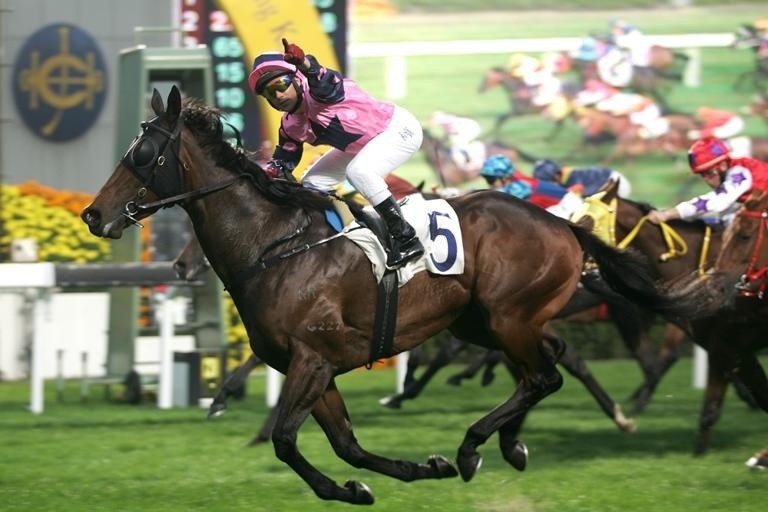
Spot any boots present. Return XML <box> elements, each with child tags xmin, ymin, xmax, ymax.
<box><xmin>373</xmin><ymin>195</ymin><xmax>424</xmax><ymax>267</ymax></box>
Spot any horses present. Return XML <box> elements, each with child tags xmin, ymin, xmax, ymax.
<box><xmin>477</xmin><ymin>32</ymin><xmax>701</xmax><ymax>166</ymax></box>
<box><xmin>731</xmin><ymin>23</ymin><xmax>768</xmax><ymax>90</ymax></box>
<box><xmin>169</xmin><ymin>230</ymin><xmax>638</xmax><ymax>452</ymax></box>
<box><xmin>739</xmin><ymin>92</ymin><xmax>768</xmax><ymax>128</ymax></box>
<box><xmin>666</xmin><ymin>122</ymin><xmax>767</xmax><ymax>166</ymax></box>
<box><xmin>380</xmin><ymin>178</ymin><xmax>768</xmax><ymax>475</ymax></box>
<box><xmin>79</xmin><ymin>83</ymin><xmax>698</xmax><ymax>506</ymax></box>
<box><xmin>418</xmin><ymin>129</ymin><xmax>531</xmax><ymax>193</ymax></box>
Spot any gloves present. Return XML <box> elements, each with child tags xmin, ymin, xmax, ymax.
<box><xmin>281</xmin><ymin>39</ymin><xmax>307</xmax><ymax>65</ymax></box>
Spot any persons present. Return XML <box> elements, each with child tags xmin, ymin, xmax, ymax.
<box><xmin>647</xmin><ymin>135</ymin><xmax>768</xmax><ymax>225</ymax></box>
<box><xmin>532</xmin><ymin>158</ymin><xmax>632</xmax><ymax>199</ymax></box>
<box><xmin>477</xmin><ymin>154</ymin><xmax>588</xmax><ymax>226</ymax></box>
<box><xmin>247</xmin><ymin>38</ymin><xmax>425</xmax><ymax>266</ymax></box>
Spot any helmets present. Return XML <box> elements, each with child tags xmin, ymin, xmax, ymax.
<box><xmin>688</xmin><ymin>135</ymin><xmax>730</xmax><ymax>176</ymax></box>
<box><xmin>247</xmin><ymin>51</ymin><xmax>304</xmax><ymax>90</ymax></box>
<box><xmin>479</xmin><ymin>153</ymin><xmax>515</xmax><ymax>178</ymax></box>
<box><xmin>532</xmin><ymin>158</ymin><xmax>561</xmax><ymax>182</ymax></box>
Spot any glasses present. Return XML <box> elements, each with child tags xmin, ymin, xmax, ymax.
<box><xmin>260</xmin><ymin>74</ymin><xmax>294</xmax><ymax>101</ymax></box>
